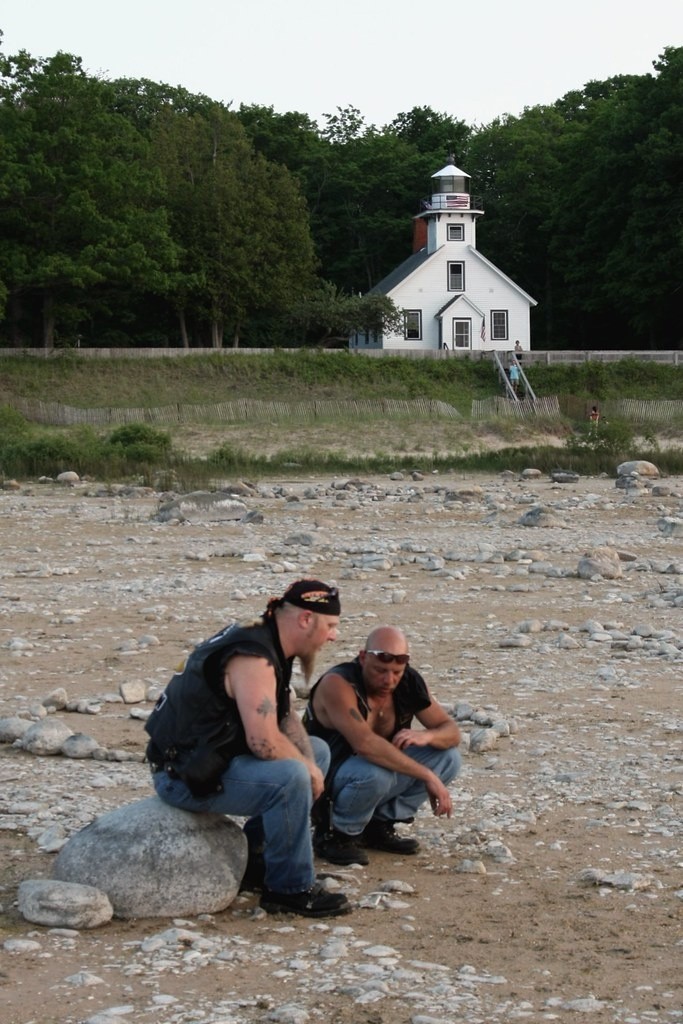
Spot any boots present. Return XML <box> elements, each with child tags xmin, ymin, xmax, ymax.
<box><xmin>351</xmin><ymin>815</ymin><xmax>421</xmax><ymax>852</ymax></box>
<box><xmin>313</xmin><ymin>827</ymin><xmax>371</xmax><ymax>865</ymax></box>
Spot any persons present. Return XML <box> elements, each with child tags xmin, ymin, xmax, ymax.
<box><xmin>141</xmin><ymin>578</ymin><xmax>352</xmax><ymax>919</ymax></box>
<box><xmin>301</xmin><ymin>625</ymin><xmax>463</xmax><ymax>867</ymax></box>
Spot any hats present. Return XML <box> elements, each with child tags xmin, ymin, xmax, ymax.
<box><xmin>285</xmin><ymin>580</ymin><xmax>341</xmax><ymax>617</ymax></box>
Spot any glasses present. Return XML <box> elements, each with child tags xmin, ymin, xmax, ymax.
<box><xmin>364</xmin><ymin>649</ymin><xmax>410</xmax><ymax>665</ymax></box>
<box><xmin>315</xmin><ymin>586</ymin><xmax>340</xmax><ymax>605</ymax></box>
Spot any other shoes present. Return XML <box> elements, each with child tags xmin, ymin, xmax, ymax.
<box><xmin>239</xmin><ymin>870</ymin><xmax>267</xmax><ymax>894</ymax></box>
<box><xmin>259</xmin><ymin>883</ymin><xmax>350</xmax><ymax>916</ymax></box>
